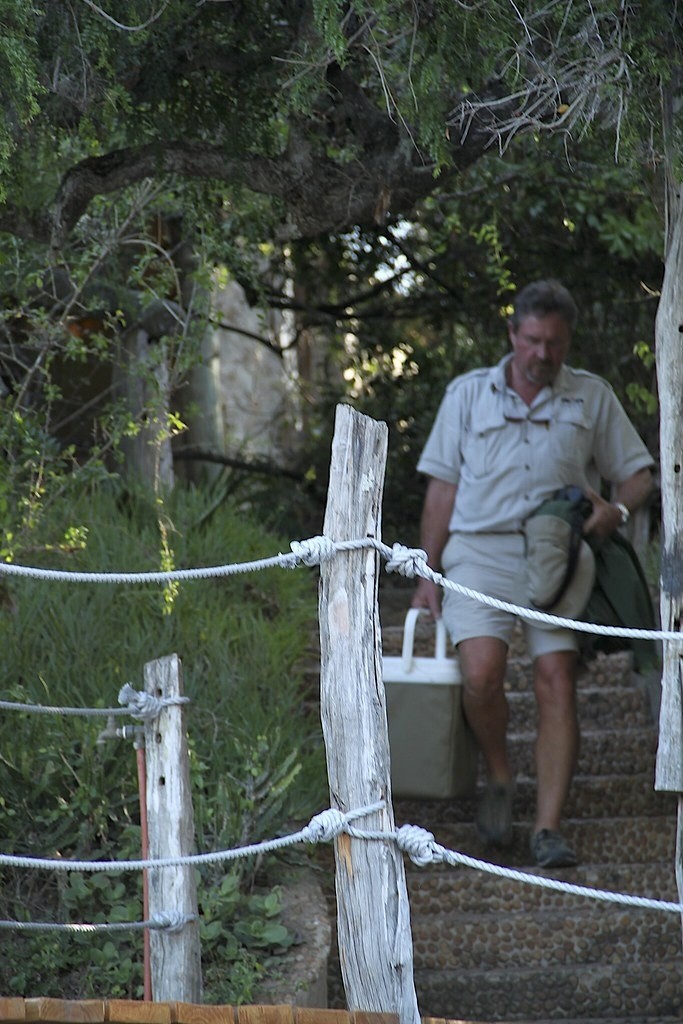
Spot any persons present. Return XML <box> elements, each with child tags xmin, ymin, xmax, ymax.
<box><xmin>410</xmin><ymin>274</ymin><xmax>655</xmax><ymax>872</ymax></box>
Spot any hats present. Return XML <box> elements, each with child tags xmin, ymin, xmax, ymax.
<box><xmin>520</xmin><ymin>515</ymin><xmax>595</xmax><ymax>630</ymax></box>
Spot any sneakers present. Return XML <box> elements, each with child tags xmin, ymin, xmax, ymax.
<box><xmin>476</xmin><ymin>774</ymin><xmax>516</xmax><ymax>851</ymax></box>
<box><xmin>529</xmin><ymin>829</ymin><xmax>576</xmax><ymax>867</ymax></box>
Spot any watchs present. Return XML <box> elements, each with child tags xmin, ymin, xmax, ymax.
<box><xmin>616</xmin><ymin>501</ymin><xmax>633</xmax><ymax>525</ymax></box>
